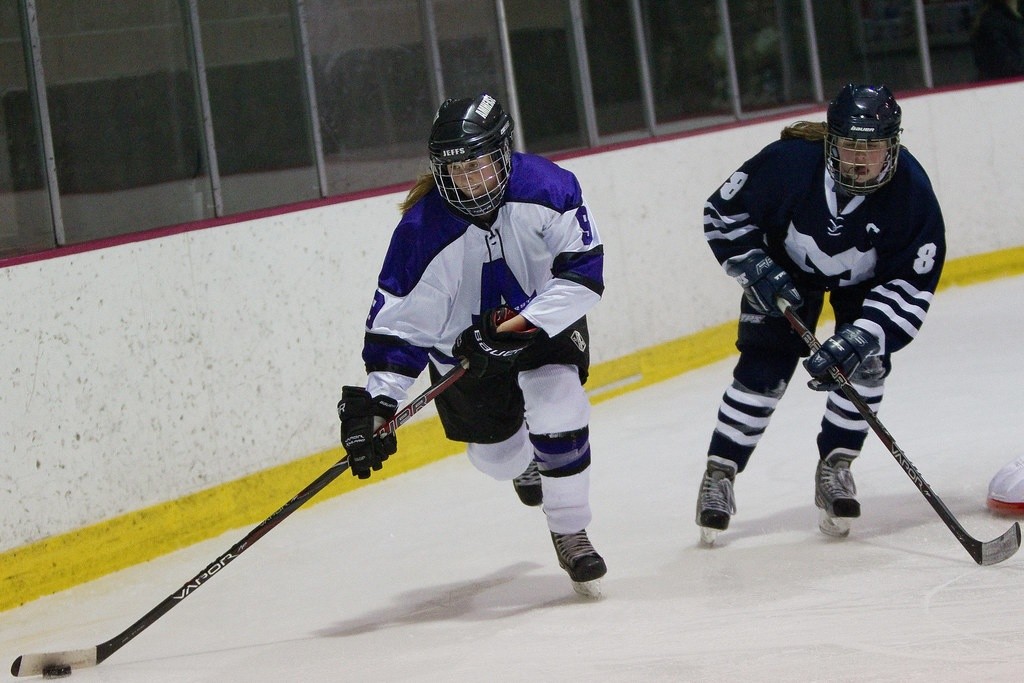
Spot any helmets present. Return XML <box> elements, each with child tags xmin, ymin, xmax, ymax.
<box><xmin>824</xmin><ymin>82</ymin><xmax>903</xmax><ymax>195</ymax></box>
<box><xmin>427</xmin><ymin>93</ymin><xmax>514</xmax><ymax>216</ymax></box>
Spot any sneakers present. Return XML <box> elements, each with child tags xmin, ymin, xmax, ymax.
<box><xmin>550</xmin><ymin>528</ymin><xmax>607</xmax><ymax>600</ymax></box>
<box><xmin>695</xmin><ymin>454</ymin><xmax>737</xmax><ymax>547</ymax></box>
<box><xmin>513</xmin><ymin>452</ymin><xmax>543</xmax><ymax>506</ymax></box>
<box><xmin>815</xmin><ymin>447</ymin><xmax>860</xmax><ymax>539</ymax></box>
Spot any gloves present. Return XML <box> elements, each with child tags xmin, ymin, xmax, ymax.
<box><xmin>337</xmin><ymin>386</ymin><xmax>397</xmax><ymax>480</ymax></box>
<box><xmin>802</xmin><ymin>323</ymin><xmax>880</xmax><ymax>391</ymax></box>
<box><xmin>452</xmin><ymin>306</ymin><xmax>542</xmax><ymax>380</ymax></box>
<box><xmin>725</xmin><ymin>253</ymin><xmax>804</xmax><ymax>317</ymax></box>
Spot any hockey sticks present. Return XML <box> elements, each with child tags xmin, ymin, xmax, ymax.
<box><xmin>777</xmin><ymin>293</ymin><xmax>1023</xmax><ymax>566</ymax></box>
<box><xmin>9</xmin><ymin>355</ymin><xmax>472</xmax><ymax>680</ymax></box>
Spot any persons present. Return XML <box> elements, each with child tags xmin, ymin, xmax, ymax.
<box><xmin>693</xmin><ymin>84</ymin><xmax>948</xmax><ymax>551</ymax></box>
<box><xmin>363</xmin><ymin>93</ymin><xmax>607</xmax><ymax>599</ymax></box>
